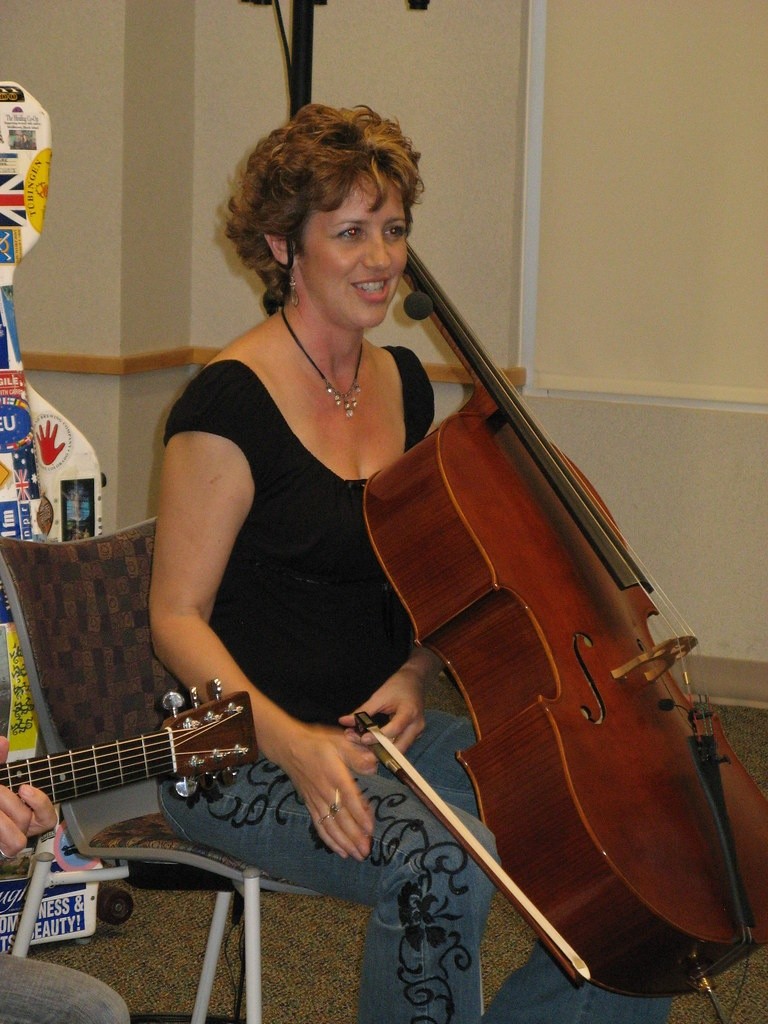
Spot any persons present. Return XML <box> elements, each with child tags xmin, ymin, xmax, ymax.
<box><xmin>0</xmin><ymin>735</ymin><xmax>131</xmax><ymax>1024</ymax></box>
<box><xmin>147</xmin><ymin>103</ymin><xmax>671</xmax><ymax>1024</ymax></box>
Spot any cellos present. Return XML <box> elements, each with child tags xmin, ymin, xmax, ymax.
<box><xmin>357</xmin><ymin>243</ymin><xmax>765</xmax><ymax>1024</ymax></box>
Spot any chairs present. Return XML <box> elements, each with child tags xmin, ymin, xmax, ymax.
<box><xmin>0</xmin><ymin>516</ymin><xmax>318</xmax><ymax>1024</ymax></box>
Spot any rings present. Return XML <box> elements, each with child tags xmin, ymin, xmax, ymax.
<box><xmin>319</xmin><ymin>814</ymin><xmax>330</xmax><ymax>823</ymax></box>
<box><xmin>329</xmin><ymin>803</ymin><xmax>341</xmax><ymax>817</ymax></box>
<box><xmin>0</xmin><ymin>850</ymin><xmax>16</xmax><ymax>864</ymax></box>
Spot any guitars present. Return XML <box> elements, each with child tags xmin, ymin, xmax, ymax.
<box><xmin>0</xmin><ymin>678</ymin><xmax>272</xmax><ymax>809</ymax></box>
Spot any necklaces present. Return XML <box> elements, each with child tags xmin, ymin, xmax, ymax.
<box><xmin>282</xmin><ymin>307</ymin><xmax>363</xmax><ymax>418</ymax></box>
